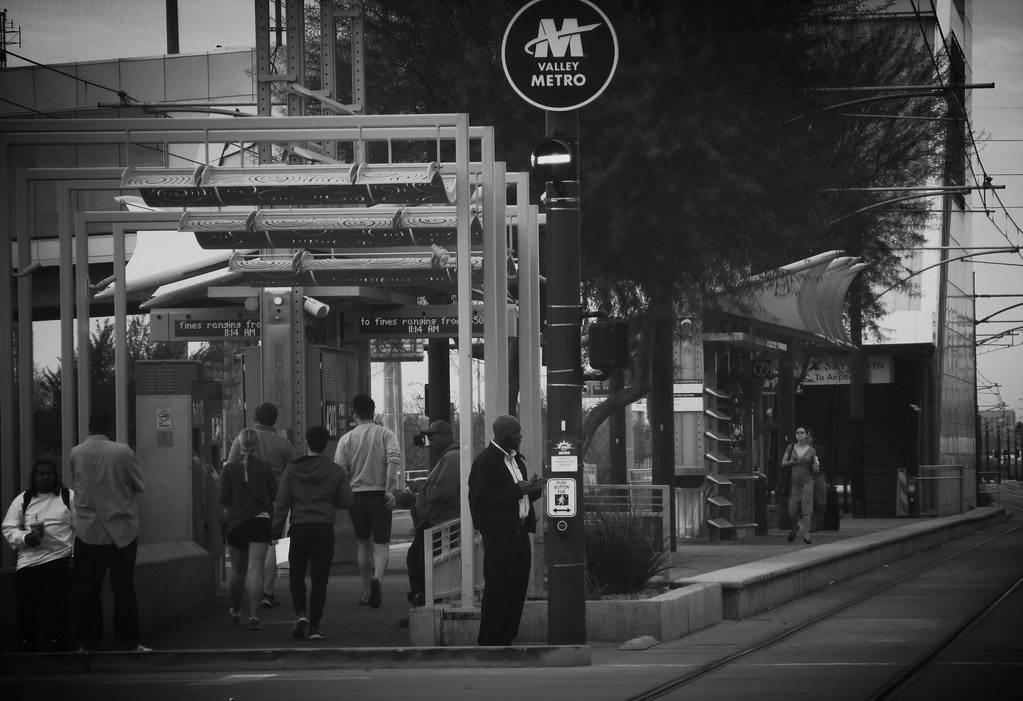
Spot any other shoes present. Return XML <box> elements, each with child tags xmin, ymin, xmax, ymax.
<box><xmin>308</xmin><ymin>627</ymin><xmax>325</xmax><ymax>639</ymax></box>
<box><xmin>358</xmin><ymin>596</ymin><xmax>368</xmax><ymax>606</ymax></box>
<box><xmin>292</xmin><ymin>613</ymin><xmax>309</xmax><ymax>638</ymax></box>
<box><xmin>137</xmin><ymin>644</ymin><xmax>152</xmax><ymax>652</ymax></box>
<box><xmin>788</xmin><ymin>531</ymin><xmax>796</xmax><ymax>542</ymax></box>
<box><xmin>369</xmin><ymin>577</ymin><xmax>381</xmax><ymax>607</ymax></box>
<box><xmin>261</xmin><ymin>592</ymin><xmax>281</xmax><ymax>608</ymax></box>
<box><xmin>804</xmin><ymin>537</ymin><xmax>810</xmax><ymax>544</ymax></box>
<box><xmin>247</xmin><ymin>616</ymin><xmax>260</xmax><ymax>631</ymax></box>
<box><xmin>399</xmin><ymin>618</ymin><xmax>409</xmax><ymax>626</ymax></box>
<box><xmin>229</xmin><ymin>608</ymin><xmax>241</xmax><ymax>624</ymax></box>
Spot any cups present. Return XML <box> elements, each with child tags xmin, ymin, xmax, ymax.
<box><xmin>29</xmin><ymin>519</ymin><xmax>45</xmax><ymax>549</ymax></box>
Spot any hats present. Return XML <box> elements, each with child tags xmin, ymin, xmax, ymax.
<box><xmin>420</xmin><ymin>420</ymin><xmax>453</xmax><ymax>434</ymax></box>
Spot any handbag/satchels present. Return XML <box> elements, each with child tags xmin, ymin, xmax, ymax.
<box><xmin>773</xmin><ymin>442</ymin><xmax>794</xmax><ymax>494</ymax></box>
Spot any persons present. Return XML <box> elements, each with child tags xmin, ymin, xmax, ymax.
<box><xmin>1</xmin><ymin>461</ymin><xmax>76</xmax><ymax>645</ymax></box>
<box><xmin>468</xmin><ymin>415</ymin><xmax>542</xmax><ymax>646</ymax></box>
<box><xmin>405</xmin><ymin>419</ymin><xmax>460</xmax><ymax>609</ymax></box>
<box><xmin>270</xmin><ymin>425</ymin><xmax>354</xmax><ymax>639</ymax></box>
<box><xmin>806</xmin><ymin>434</ymin><xmax>826</xmax><ymax>532</ymax></box>
<box><xmin>335</xmin><ymin>395</ymin><xmax>401</xmax><ymax>608</ymax></box>
<box><xmin>70</xmin><ymin>407</ymin><xmax>153</xmax><ymax>653</ymax></box>
<box><xmin>782</xmin><ymin>427</ymin><xmax>820</xmax><ymax>544</ymax></box>
<box><xmin>218</xmin><ymin>428</ymin><xmax>279</xmax><ymax>629</ymax></box>
<box><xmin>227</xmin><ymin>403</ymin><xmax>299</xmax><ymax>610</ymax></box>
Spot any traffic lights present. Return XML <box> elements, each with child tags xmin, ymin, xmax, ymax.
<box><xmin>589</xmin><ymin>318</ymin><xmax>629</xmax><ymax>369</ymax></box>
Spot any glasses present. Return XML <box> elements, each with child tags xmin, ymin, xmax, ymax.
<box><xmin>796</xmin><ymin>431</ymin><xmax>805</xmax><ymax>434</ymax></box>
